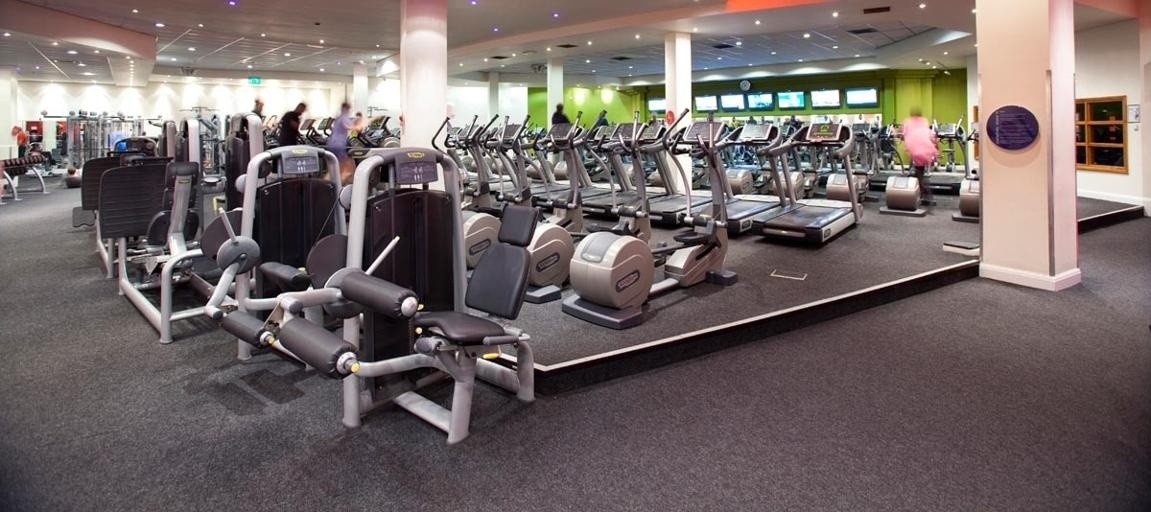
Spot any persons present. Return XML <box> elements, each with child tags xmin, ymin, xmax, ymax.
<box><xmin>664</xmin><ymin>111</ymin><xmax>677</xmax><ymax>137</ymax></box>
<box><xmin>707</xmin><ymin>112</ymin><xmax>713</xmax><ymax>122</ymax></box>
<box><xmin>551</xmin><ymin>103</ymin><xmax>573</xmax><ymax>124</ymax></box>
<box><xmin>903</xmin><ymin>105</ymin><xmax>940</xmax><ymax>201</ymax></box>
<box><xmin>251</xmin><ymin>98</ymin><xmax>365</xmax><ymax>157</ymax></box>
<box><xmin>594</xmin><ymin>110</ymin><xmax>611</xmax><ymax>127</ymax></box>
<box><xmin>732</xmin><ymin>109</ymin><xmax>881</xmax><ymax>126</ymax></box>
<box><xmin>10</xmin><ymin>126</ymin><xmax>27</xmax><ymax>158</ymax></box>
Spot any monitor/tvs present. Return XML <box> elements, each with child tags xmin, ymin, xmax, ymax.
<box><xmin>746</xmin><ymin>91</ymin><xmax>775</xmax><ymax>112</ymax></box>
<box><xmin>318</xmin><ymin>118</ymin><xmax>334</xmax><ymax>130</ymax></box>
<box><xmin>805</xmin><ymin>122</ymin><xmax>843</xmax><ymax>142</ymax></box>
<box><xmin>719</xmin><ymin>91</ymin><xmax>747</xmax><ymax>112</ymax></box>
<box><xmin>694</xmin><ymin>93</ymin><xmax>719</xmax><ymax>112</ymax></box>
<box><xmin>777</xmin><ymin>90</ymin><xmax>806</xmax><ymax>110</ymax></box>
<box><xmin>852</xmin><ymin>123</ymin><xmax>871</xmax><ymax>134</ymax></box>
<box><xmin>369</xmin><ymin>115</ymin><xmax>390</xmax><ymax>130</ymax></box>
<box><xmin>809</xmin><ymin>88</ymin><xmax>842</xmax><ymax>110</ymax></box>
<box><xmin>845</xmin><ymin>86</ymin><xmax>880</xmax><ymax>108</ymax></box>
<box><xmin>301</xmin><ymin>119</ymin><xmax>315</xmax><ymax>130</ymax></box>
<box><xmin>648</xmin><ymin>96</ymin><xmax>666</xmax><ymax>114</ymax></box>
<box><xmin>448</xmin><ymin>121</ymin><xmax>725</xmax><ymax>142</ymax></box>
<box><xmin>738</xmin><ymin>123</ymin><xmax>773</xmax><ymax>141</ymax></box>
